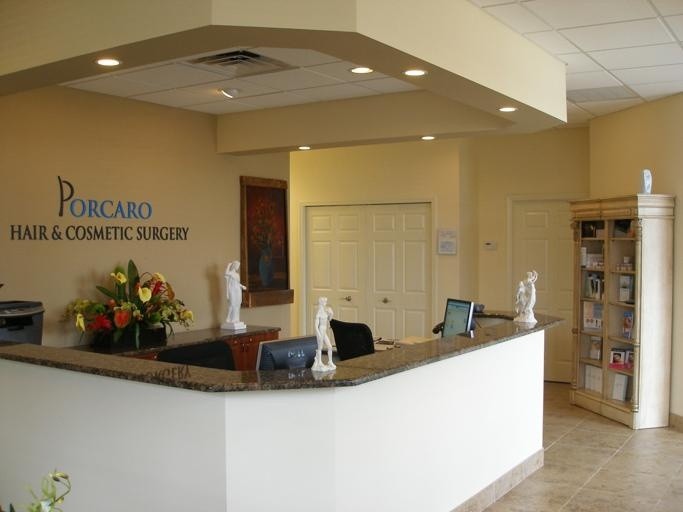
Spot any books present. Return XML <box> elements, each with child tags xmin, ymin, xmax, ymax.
<box><xmin>579</xmin><ymin>299</ymin><xmax>634</xmax><ymax>403</ymax></box>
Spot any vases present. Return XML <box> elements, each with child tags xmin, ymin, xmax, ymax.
<box><xmin>94</xmin><ymin>323</ymin><xmax>168</xmax><ymax>352</ymax></box>
<box><xmin>639</xmin><ymin>169</ymin><xmax>653</xmax><ymax>194</ymax></box>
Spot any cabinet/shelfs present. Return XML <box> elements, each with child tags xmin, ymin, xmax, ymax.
<box><xmin>566</xmin><ymin>194</ymin><xmax>677</xmax><ymax>430</ymax></box>
<box><xmin>114</xmin><ymin>331</ymin><xmax>279</xmax><ymax>371</ymax></box>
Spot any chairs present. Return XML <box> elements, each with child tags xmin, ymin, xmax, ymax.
<box><xmin>330</xmin><ymin>318</ymin><xmax>375</xmax><ymax>361</ymax></box>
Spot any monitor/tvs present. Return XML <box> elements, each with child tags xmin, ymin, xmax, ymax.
<box><xmin>441</xmin><ymin>298</ymin><xmax>475</xmax><ymax>337</ymax></box>
<box><xmin>255</xmin><ymin>335</ymin><xmax>319</xmax><ymax>370</ymax></box>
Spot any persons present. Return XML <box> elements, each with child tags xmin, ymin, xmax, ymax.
<box><xmin>310</xmin><ymin>296</ymin><xmax>337</xmax><ymax>371</ymax></box>
<box><xmin>513</xmin><ymin>270</ymin><xmax>539</xmax><ymax>323</ymax></box>
<box><xmin>223</xmin><ymin>258</ymin><xmax>246</xmax><ymax>323</ymax></box>
<box><xmin>515</xmin><ymin>279</ymin><xmax>524</xmax><ymax>315</ymax></box>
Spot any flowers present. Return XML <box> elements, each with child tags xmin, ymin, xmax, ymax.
<box><xmin>0</xmin><ymin>467</ymin><xmax>73</xmax><ymax>512</ymax></box>
<box><xmin>56</xmin><ymin>258</ymin><xmax>195</xmax><ymax>351</ymax></box>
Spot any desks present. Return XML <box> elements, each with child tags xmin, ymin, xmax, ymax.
<box><xmin>322</xmin><ymin>335</ymin><xmax>400</xmax><ymax>356</ymax></box>
